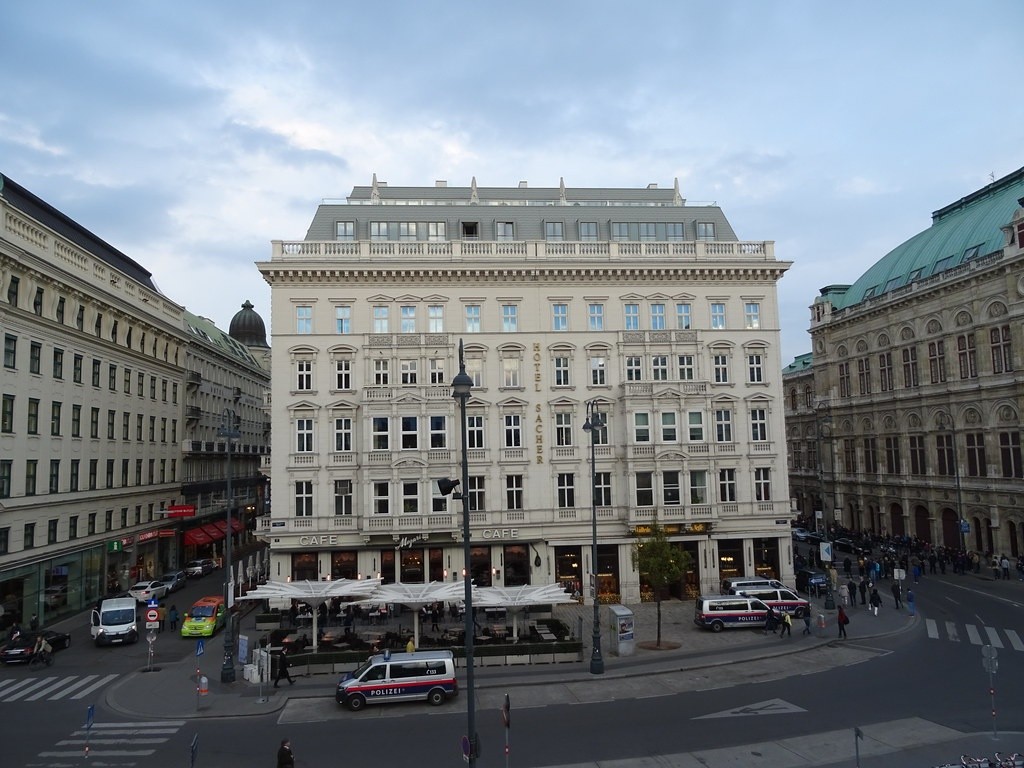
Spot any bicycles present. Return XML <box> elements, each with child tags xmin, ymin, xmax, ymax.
<box><xmin>936</xmin><ymin>752</ymin><xmax>1024</xmax><ymax>768</ymax></box>
<box><xmin>29</xmin><ymin>652</ymin><xmax>55</xmax><ymax>671</ymax></box>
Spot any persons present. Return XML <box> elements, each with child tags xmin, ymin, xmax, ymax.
<box><xmin>472</xmin><ymin>607</ymin><xmax>481</xmax><ymax>629</ymax></box>
<box><xmin>10</xmin><ymin>621</ymin><xmax>21</xmax><ymax>640</ymax></box>
<box><xmin>837</xmin><ymin>605</ymin><xmax>848</xmax><ymax>639</ymax></box>
<box><xmin>277</xmin><ymin>738</ymin><xmax>294</xmax><ymax>768</ymax></box>
<box><xmin>643</xmin><ymin>582</ymin><xmax>649</xmax><ymax>593</ymax></box>
<box><xmin>859</xmin><ymin>579</ymin><xmax>867</xmax><ymax>605</ymax></box>
<box><xmin>114</xmin><ymin>581</ymin><xmax>120</xmax><ymax>593</ymax></box>
<box><xmin>273</xmin><ymin>646</ymin><xmax>296</xmax><ymax>688</ymax></box>
<box><xmin>802</xmin><ymin>607</ymin><xmax>812</xmax><ymax>634</ymax></box>
<box><xmin>991</xmin><ymin>553</ymin><xmax>1010</xmax><ymax>580</ymax></box>
<box><xmin>158</xmin><ymin>603</ymin><xmax>167</xmax><ymax>633</ymax></box>
<box><xmin>619</xmin><ymin>623</ymin><xmax>629</xmax><ymax>634</ymax></box>
<box><xmin>765</xmin><ymin>606</ymin><xmax>777</xmax><ymax>634</ymax></box>
<box><xmin>791</xmin><ymin>514</ymin><xmax>983</xmax><ymax>585</ymax></box>
<box><xmin>406</xmin><ymin>636</ymin><xmax>415</xmax><ymax>652</ymax></box>
<box><xmin>432</xmin><ymin>607</ymin><xmax>441</xmax><ymax>632</ymax></box>
<box><xmin>33</xmin><ymin>635</ymin><xmax>52</xmax><ymax>662</ymax></box>
<box><xmin>907</xmin><ymin>588</ymin><xmax>915</xmax><ymax>617</ymax></box>
<box><xmin>891</xmin><ymin>581</ymin><xmax>904</xmax><ymax>609</ymax></box>
<box><xmin>30</xmin><ymin>613</ymin><xmax>38</xmax><ymax>631</ymax></box>
<box><xmin>561</xmin><ymin>580</ymin><xmax>579</xmax><ymax>592</ymax></box>
<box><xmin>169</xmin><ymin>605</ymin><xmax>179</xmax><ymax>632</ymax></box>
<box><xmin>779</xmin><ymin>610</ymin><xmax>792</xmax><ymax>638</ymax></box>
<box><xmin>869</xmin><ymin>588</ymin><xmax>883</xmax><ymax>617</ymax></box>
<box><xmin>838</xmin><ymin>579</ymin><xmax>857</xmax><ymax>607</ymax></box>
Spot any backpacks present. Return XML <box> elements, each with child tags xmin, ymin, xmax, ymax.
<box><xmin>992</xmin><ymin>560</ymin><xmax>997</xmax><ymax>566</ymax></box>
<box><xmin>766</xmin><ymin>613</ymin><xmax>772</xmax><ymax>620</ymax></box>
<box><xmin>779</xmin><ymin>614</ymin><xmax>788</xmax><ymax>624</ymax></box>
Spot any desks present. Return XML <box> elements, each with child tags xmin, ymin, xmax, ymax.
<box><xmin>321</xmin><ymin>630</ymin><xmax>341</xmax><ymax>641</ymax></box>
<box><xmin>537</xmin><ymin>630</ymin><xmax>550</xmax><ymax>634</ymax></box>
<box><xmin>534</xmin><ymin>625</ymin><xmax>547</xmax><ymax>628</ymax></box>
<box><xmin>536</xmin><ymin>627</ymin><xmax>549</xmax><ymax>630</ymax></box>
<box><xmin>493</xmin><ymin>627</ymin><xmax>506</xmax><ymax>630</ymax></box>
<box><xmin>281</xmin><ymin>634</ymin><xmax>301</xmax><ymax>642</ymax></box>
<box><xmin>336</xmin><ymin>614</ymin><xmax>347</xmax><ymax>626</ymax></box>
<box><xmin>333</xmin><ymin>642</ymin><xmax>350</xmax><ymax>648</ymax></box>
<box><xmin>449</xmin><ymin>628</ymin><xmax>464</xmax><ymax>634</ymax></box>
<box><xmin>368</xmin><ymin>612</ymin><xmax>381</xmax><ymax>625</ymax></box>
<box><xmin>296</xmin><ymin>614</ymin><xmax>320</xmax><ymax>627</ymax></box>
<box><xmin>505</xmin><ymin>637</ymin><xmax>519</xmax><ymax>641</ymax></box>
<box><xmin>361</xmin><ymin>630</ymin><xmax>387</xmax><ymax>640</ymax></box>
<box><xmin>304</xmin><ymin>646</ymin><xmax>319</xmax><ymax>649</ymax></box>
<box><xmin>496</xmin><ymin>630</ymin><xmax>509</xmax><ymax>634</ymax></box>
<box><xmin>485</xmin><ymin>608</ymin><xmax>507</xmax><ymax>612</ymax></box>
<box><xmin>541</xmin><ymin>634</ymin><xmax>557</xmax><ymax>640</ymax></box>
<box><xmin>475</xmin><ymin>636</ymin><xmax>493</xmax><ymax>641</ymax></box>
<box><xmin>493</xmin><ymin>624</ymin><xmax>507</xmax><ymax>628</ymax></box>
<box><xmin>400</xmin><ymin>633</ymin><xmax>414</xmax><ymax>638</ymax></box>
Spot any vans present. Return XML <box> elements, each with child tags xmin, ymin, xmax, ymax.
<box><xmin>335</xmin><ymin>649</ymin><xmax>458</xmax><ymax>712</ymax></box>
<box><xmin>693</xmin><ymin>596</ymin><xmax>782</xmax><ymax>632</ymax></box>
<box><xmin>796</xmin><ymin>567</ymin><xmax>830</xmax><ymax>594</ymax></box>
<box><xmin>182</xmin><ymin>597</ymin><xmax>227</xmax><ymax>638</ymax></box>
<box><xmin>721</xmin><ymin>576</ymin><xmax>797</xmax><ymax>597</ymax></box>
<box><xmin>92</xmin><ymin>597</ymin><xmax>141</xmax><ymax>647</ymax></box>
<box><xmin>730</xmin><ymin>586</ymin><xmax>812</xmax><ymax>618</ymax></box>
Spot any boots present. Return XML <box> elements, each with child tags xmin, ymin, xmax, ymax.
<box><xmin>802</xmin><ymin>627</ymin><xmax>811</xmax><ymax>635</ymax></box>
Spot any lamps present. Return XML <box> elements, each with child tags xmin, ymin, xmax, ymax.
<box><xmin>377</xmin><ymin>572</ymin><xmax>381</xmax><ymax>578</ymax></box>
<box><xmin>493</xmin><ymin>567</ymin><xmax>496</xmax><ymax>576</ymax></box>
<box><xmin>358</xmin><ymin>573</ymin><xmax>362</xmax><ymax>580</ymax></box>
<box><xmin>287</xmin><ymin>576</ymin><xmax>290</xmax><ymax>583</ymax></box>
<box><xmin>327</xmin><ymin>574</ymin><xmax>330</xmax><ymax>581</ymax></box>
<box><xmin>444</xmin><ymin>569</ymin><xmax>448</xmax><ymax>578</ymax></box>
<box><xmin>462</xmin><ymin>568</ymin><xmax>466</xmax><ymax>577</ymax></box>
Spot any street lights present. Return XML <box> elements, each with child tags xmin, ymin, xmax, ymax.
<box><xmin>582</xmin><ymin>400</ymin><xmax>607</xmax><ymax>673</ymax></box>
<box><xmin>938</xmin><ymin>412</ymin><xmax>970</xmax><ymax>569</ymax></box>
<box><xmin>814</xmin><ymin>402</ymin><xmax>835</xmax><ymax>540</ymax></box>
<box><xmin>220</xmin><ymin>408</ymin><xmax>242</xmax><ymax>684</ymax></box>
<box><xmin>450</xmin><ymin>343</ymin><xmax>477</xmax><ymax>768</ymax></box>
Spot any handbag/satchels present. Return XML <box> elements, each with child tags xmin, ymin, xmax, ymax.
<box><xmin>844</xmin><ymin>616</ymin><xmax>849</xmax><ymax>625</ymax></box>
<box><xmin>868</xmin><ymin>603</ymin><xmax>871</xmax><ymax>611</ymax></box>
<box><xmin>176</xmin><ymin>616</ymin><xmax>179</xmax><ymax>621</ymax></box>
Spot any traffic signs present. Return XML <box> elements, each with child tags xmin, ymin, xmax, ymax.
<box><xmin>808</xmin><ymin>578</ymin><xmax>824</xmax><ymax>585</ymax></box>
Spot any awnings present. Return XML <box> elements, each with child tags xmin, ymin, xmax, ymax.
<box><xmin>184</xmin><ymin>516</ymin><xmax>245</xmax><ymax>546</ymax></box>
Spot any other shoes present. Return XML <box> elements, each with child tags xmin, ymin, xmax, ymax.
<box><xmin>290</xmin><ymin>679</ymin><xmax>296</xmax><ymax>685</ymax></box>
<box><xmin>773</xmin><ymin>631</ymin><xmax>777</xmax><ymax>633</ymax></box>
<box><xmin>780</xmin><ymin>634</ymin><xmax>782</xmax><ymax>638</ymax></box>
<box><xmin>274</xmin><ymin>685</ymin><xmax>280</xmax><ymax>688</ymax></box>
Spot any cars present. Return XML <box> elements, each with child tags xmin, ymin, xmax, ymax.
<box><xmin>129</xmin><ymin>559</ymin><xmax>213</xmax><ymax>604</ymax></box>
<box><xmin>834</xmin><ymin>537</ymin><xmax>867</xmax><ymax>555</ymax></box>
<box><xmin>0</xmin><ymin>630</ymin><xmax>71</xmax><ymax>664</ymax></box>
<box><xmin>793</xmin><ymin>527</ymin><xmax>807</xmax><ymax>541</ymax></box>
<box><xmin>805</xmin><ymin>531</ymin><xmax>835</xmax><ymax>545</ymax></box>
